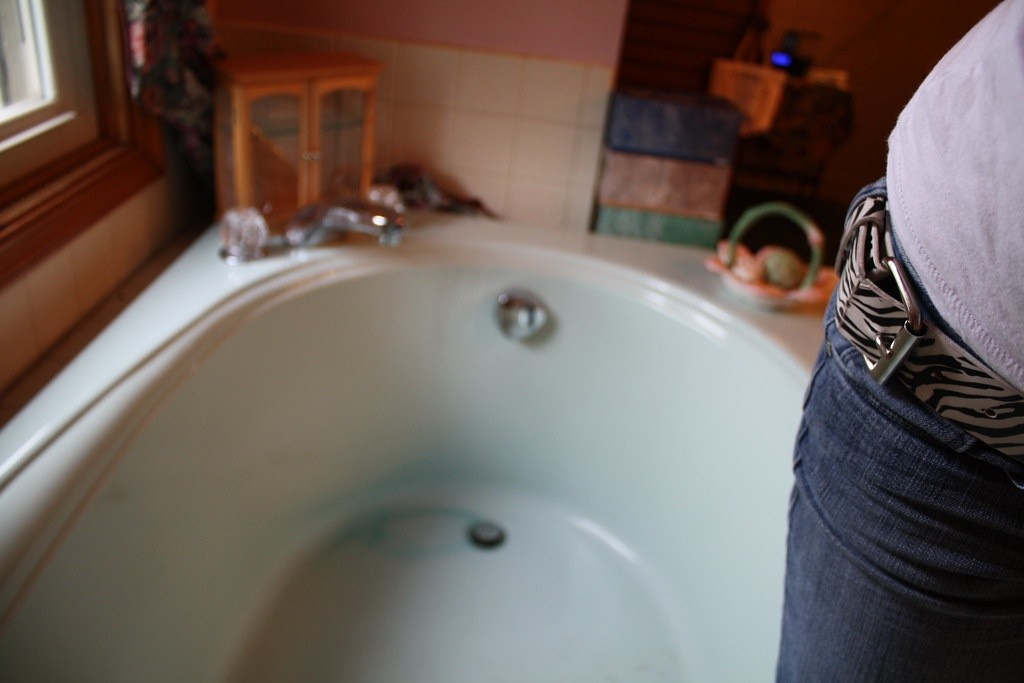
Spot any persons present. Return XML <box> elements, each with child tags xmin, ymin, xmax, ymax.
<box><xmin>774</xmin><ymin>0</ymin><xmax>1024</xmax><ymax>683</ymax></box>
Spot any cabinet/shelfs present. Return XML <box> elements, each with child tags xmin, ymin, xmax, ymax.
<box><xmin>211</xmin><ymin>46</ymin><xmax>387</xmax><ymax>237</ymax></box>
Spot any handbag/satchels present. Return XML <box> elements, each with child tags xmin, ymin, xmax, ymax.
<box><xmin>710</xmin><ymin>25</ymin><xmax>788</xmax><ymax>139</ymax></box>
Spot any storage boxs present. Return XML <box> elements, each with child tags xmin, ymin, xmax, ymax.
<box><xmin>593</xmin><ymin>85</ymin><xmax>748</xmax><ymax>251</ymax></box>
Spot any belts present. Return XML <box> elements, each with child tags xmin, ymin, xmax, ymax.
<box><xmin>834</xmin><ymin>196</ymin><xmax>1024</xmax><ymax>461</ymax></box>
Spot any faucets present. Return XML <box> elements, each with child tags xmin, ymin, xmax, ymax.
<box><xmin>293</xmin><ymin>200</ymin><xmax>406</xmax><ymax>254</ymax></box>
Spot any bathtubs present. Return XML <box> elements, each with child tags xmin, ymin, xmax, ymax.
<box><xmin>0</xmin><ymin>215</ymin><xmax>839</xmax><ymax>683</ymax></box>
